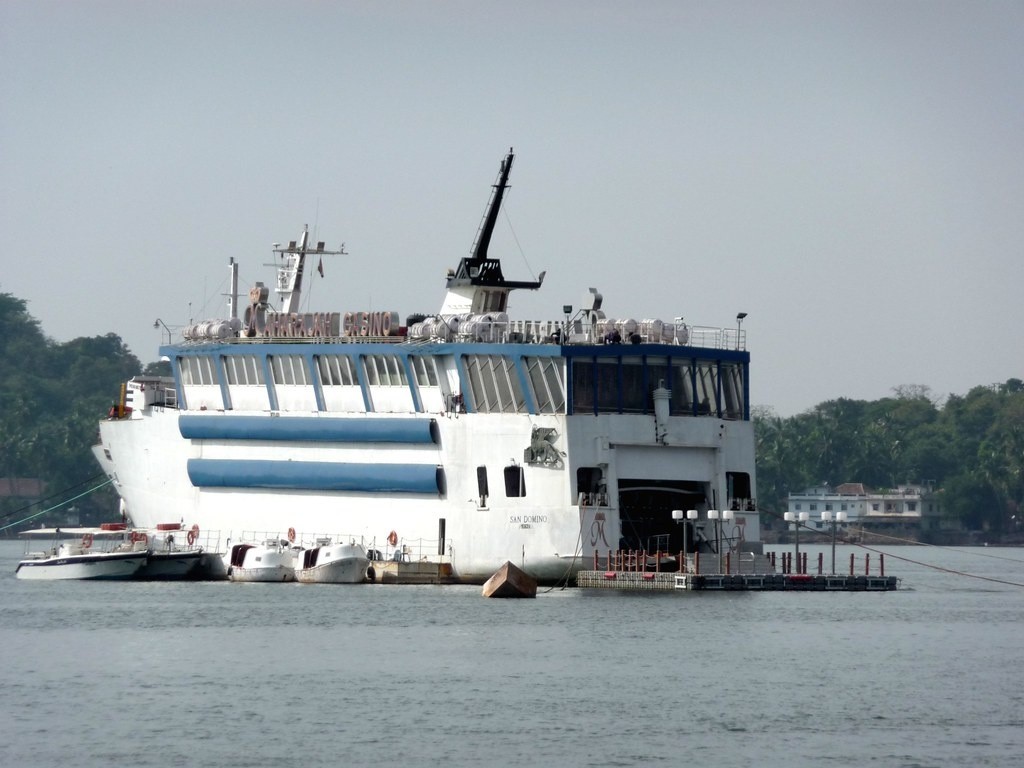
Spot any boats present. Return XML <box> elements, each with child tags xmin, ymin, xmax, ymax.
<box><xmin>293</xmin><ymin>541</ymin><xmax>372</xmax><ymax>585</ymax></box>
<box><xmin>129</xmin><ymin>522</ymin><xmax>224</xmax><ymax>578</ymax></box>
<box><xmin>91</xmin><ymin>148</ymin><xmax>760</xmax><ymax>585</ymax></box>
<box><xmin>14</xmin><ymin>523</ymin><xmax>150</xmax><ymax>582</ymax></box>
<box><xmin>221</xmin><ymin>536</ymin><xmax>296</xmax><ymax>584</ymax></box>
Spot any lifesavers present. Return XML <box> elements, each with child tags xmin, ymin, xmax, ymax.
<box><xmin>131</xmin><ymin>532</ymin><xmax>148</xmax><ymax>545</ymax></box>
<box><xmin>410</xmin><ymin>312</ymin><xmax>508</xmax><ymax>342</ymax></box>
<box><xmin>287</xmin><ymin>527</ymin><xmax>295</xmax><ymax>541</ymax></box>
<box><xmin>597</xmin><ymin>319</ymin><xmax>689</xmax><ymax>343</ymax></box>
<box><xmin>192</xmin><ymin>524</ymin><xmax>199</xmax><ymax>538</ymax></box>
<box><xmin>187</xmin><ymin>531</ymin><xmax>194</xmax><ymax>544</ymax></box>
<box><xmin>82</xmin><ymin>535</ymin><xmax>92</xmax><ymax>547</ymax></box>
<box><xmin>389</xmin><ymin>530</ymin><xmax>397</xmax><ymax>545</ymax></box>
